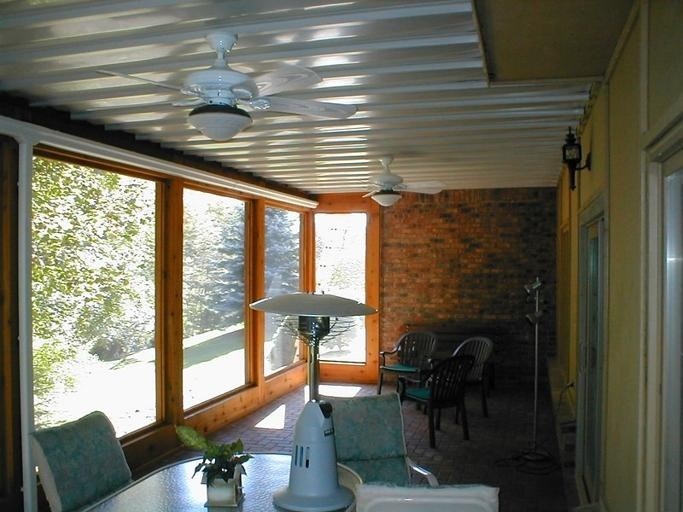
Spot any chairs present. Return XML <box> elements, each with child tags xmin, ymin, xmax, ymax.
<box><xmin>29</xmin><ymin>410</ymin><xmax>133</xmax><ymax>512</ymax></box>
<box><xmin>315</xmin><ymin>391</ymin><xmax>440</xmax><ymax>500</ymax></box>
<box><xmin>378</xmin><ymin>332</ymin><xmax>496</xmax><ymax>449</ymax></box>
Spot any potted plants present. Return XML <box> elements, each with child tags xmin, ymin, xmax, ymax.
<box><xmin>173</xmin><ymin>425</ymin><xmax>254</xmax><ymax>508</ymax></box>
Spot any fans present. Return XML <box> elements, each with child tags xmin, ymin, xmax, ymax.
<box><xmin>100</xmin><ymin>33</ymin><xmax>358</xmax><ymax>143</ymax></box>
<box><xmin>361</xmin><ymin>156</ymin><xmax>444</xmax><ymax>208</ymax></box>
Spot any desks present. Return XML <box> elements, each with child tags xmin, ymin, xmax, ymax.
<box><xmin>80</xmin><ymin>453</ymin><xmax>362</xmax><ymax>512</ymax></box>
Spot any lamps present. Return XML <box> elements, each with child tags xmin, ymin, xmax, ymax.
<box><xmin>189</xmin><ymin>103</ymin><xmax>252</xmax><ymax>142</ymax></box>
<box><xmin>249</xmin><ymin>293</ymin><xmax>380</xmax><ymax>512</ymax></box>
<box><xmin>370</xmin><ymin>190</ymin><xmax>403</xmax><ymax>207</ymax></box>
<box><xmin>515</xmin><ymin>276</ymin><xmax>549</xmax><ymax>462</ymax></box>
<box><xmin>562</xmin><ymin>126</ymin><xmax>593</xmax><ymax>190</ymax></box>
